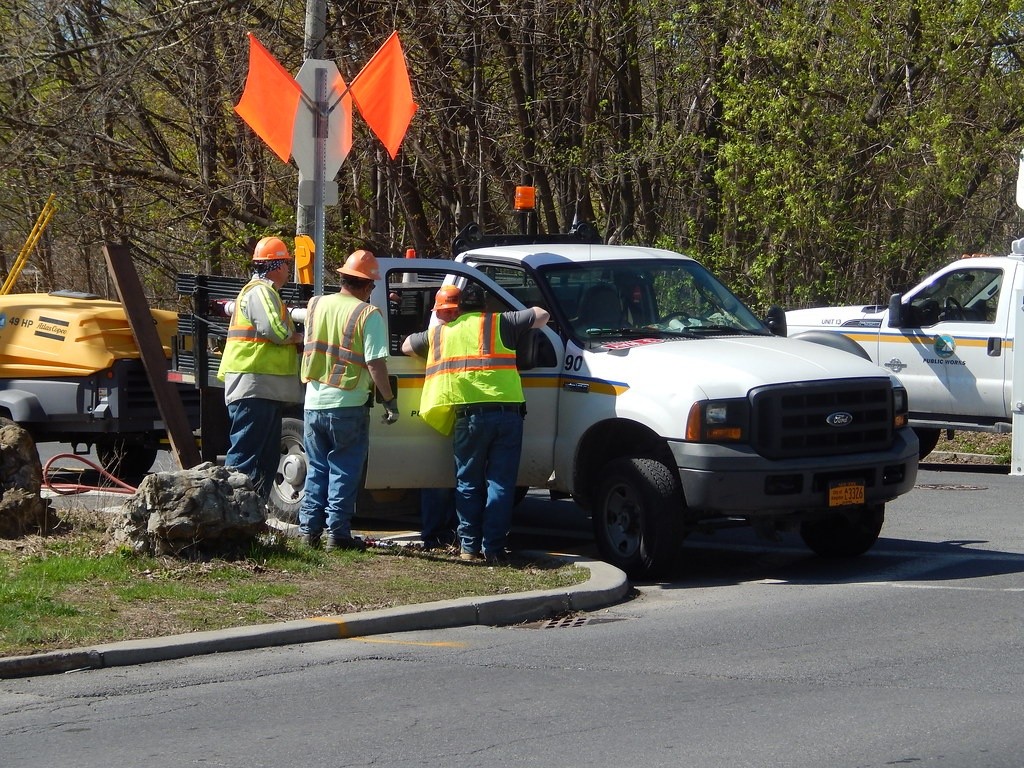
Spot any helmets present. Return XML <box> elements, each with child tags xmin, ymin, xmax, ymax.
<box><xmin>336</xmin><ymin>249</ymin><xmax>381</xmax><ymax>280</ymax></box>
<box><xmin>431</xmin><ymin>286</ymin><xmax>462</xmax><ymax>312</ymax></box>
<box><xmin>253</xmin><ymin>236</ymin><xmax>293</xmax><ymax>260</ymax></box>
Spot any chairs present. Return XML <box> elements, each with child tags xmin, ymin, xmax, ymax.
<box><xmin>571</xmin><ymin>285</ymin><xmax>632</xmax><ymax>335</ymax></box>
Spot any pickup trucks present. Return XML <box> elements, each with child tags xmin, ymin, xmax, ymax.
<box><xmin>165</xmin><ymin>222</ymin><xmax>920</xmax><ymax>582</ymax></box>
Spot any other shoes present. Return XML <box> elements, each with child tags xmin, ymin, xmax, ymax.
<box><xmin>485</xmin><ymin>552</ymin><xmax>511</xmax><ymax>563</ymax></box>
<box><xmin>300</xmin><ymin>531</ymin><xmax>323</xmax><ymax>551</ymax></box>
<box><xmin>460</xmin><ymin>547</ymin><xmax>479</xmax><ymax>560</ymax></box>
<box><xmin>325</xmin><ymin>532</ymin><xmax>367</xmax><ymax>554</ymax></box>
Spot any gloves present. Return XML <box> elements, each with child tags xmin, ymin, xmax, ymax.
<box><xmin>381</xmin><ymin>397</ymin><xmax>400</xmax><ymax>425</ymax></box>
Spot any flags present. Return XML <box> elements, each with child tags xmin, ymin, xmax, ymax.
<box><xmin>346</xmin><ymin>29</ymin><xmax>419</xmax><ymax>159</ymax></box>
<box><xmin>232</xmin><ymin>32</ymin><xmax>304</xmax><ymax>165</ymax></box>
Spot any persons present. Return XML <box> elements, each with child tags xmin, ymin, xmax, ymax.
<box><xmin>299</xmin><ymin>249</ymin><xmax>400</xmax><ymax>552</ymax></box>
<box><xmin>401</xmin><ymin>284</ymin><xmax>551</xmax><ymax>563</ymax></box>
<box><xmin>216</xmin><ymin>236</ymin><xmax>304</xmax><ymax>503</ymax></box>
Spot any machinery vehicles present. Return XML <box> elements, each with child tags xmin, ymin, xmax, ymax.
<box><xmin>0</xmin><ymin>183</ymin><xmax>200</xmax><ymax>486</ymax></box>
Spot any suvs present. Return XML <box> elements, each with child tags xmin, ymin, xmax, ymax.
<box><xmin>785</xmin><ymin>234</ymin><xmax>1023</xmax><ymax>458</ymax></box>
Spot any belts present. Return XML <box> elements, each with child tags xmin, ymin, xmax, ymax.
<box><xmin>456</xmin><ymin>403</ymin><xmax>523</xmax><ymax>419</ymax></box>
